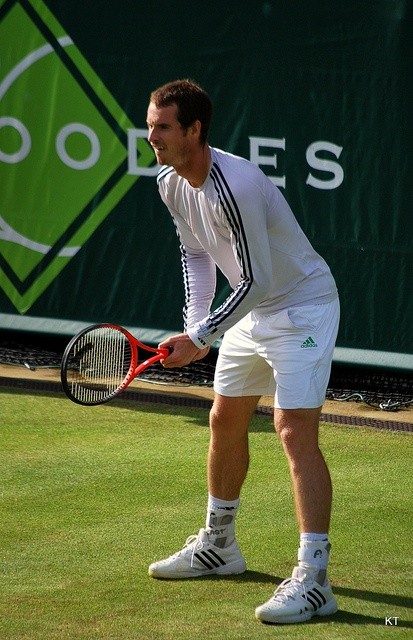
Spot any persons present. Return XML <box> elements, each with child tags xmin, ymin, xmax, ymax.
<box><xmin>145</xmin><ymin>79</ymin><xmax>343</xmax><ymax>625</ymax></box>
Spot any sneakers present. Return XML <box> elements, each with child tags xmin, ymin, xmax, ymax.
<box><xmin>149</xmin><ymin>529</ymin><xmax>246</xmax><ymax>579</ymax></box>
<box><xmin>254</xmin><ymin>569</ymin><xmax>338</xmax><ymax>624</ymax></box>
